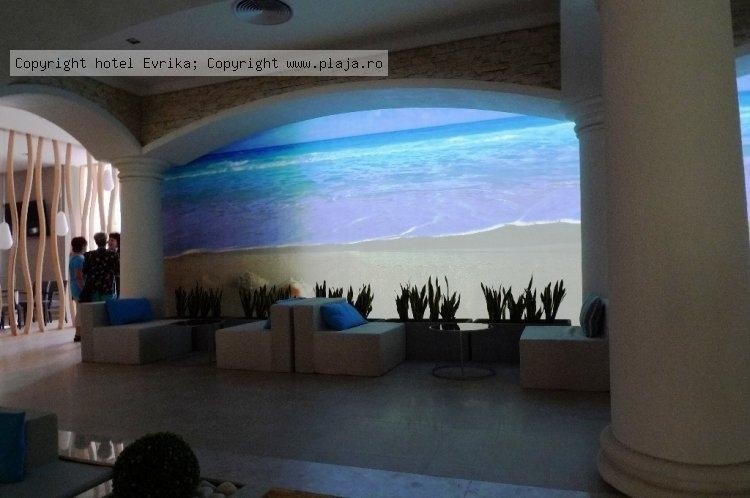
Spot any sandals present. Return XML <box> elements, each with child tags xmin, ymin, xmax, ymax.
<box><xmin>74</xmin><ymin>334</ymin><xmax>81</xmax><ymax>342</ymax></box>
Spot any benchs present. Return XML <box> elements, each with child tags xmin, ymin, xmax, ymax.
<box><xmin>292</xmin><ymin>295</ymin><xmax>406</xmax><ymax>376</ymax></box>
<box><xmin>78</xmin><ymin>299</ymin><xmax>193</xmax><ymax>366</ymax></box>
<box><xmin>214</xmin><ymin>295</ymin><xmax>301</xmax><ymax>373</ymax></box>
<box><xmin>520</xmin><ymin>295</ymin><xmax>610</xmax><ymax>391</ymax></box>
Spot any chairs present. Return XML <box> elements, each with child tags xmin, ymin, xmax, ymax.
<box><xmin>1</xmin><ymin>278</ymin><xmax>67</xmax><ymax>333</ymax></box>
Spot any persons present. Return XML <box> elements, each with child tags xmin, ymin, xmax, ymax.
<box><xmin>105</xmin><ymin>232</ymin><xmax>121</xmax><ymax>302</ymax></box>
<box><xmin>68</xmin><ymin>235</ymin><xmax>89</xmax><ymax>343</ymax></box>
<box><xmin>80</xmin><ymin>232</ymin><xmax>121</xmax><ymax>342</ymax></box>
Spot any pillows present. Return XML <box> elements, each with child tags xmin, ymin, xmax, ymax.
<box><xmin>106</xmin><ymin>298</ymin><xmax>152</xmax><ymax>326</ymax></box>
<box><xmin>321</xmin><ymin>300</ymin><xmax>368</xmax><ymax>331</ymax></box>
<box><xmin>579</xmin><ymin>296</ymin><xmax>603</xmax><ymax>338</ymax></box>
